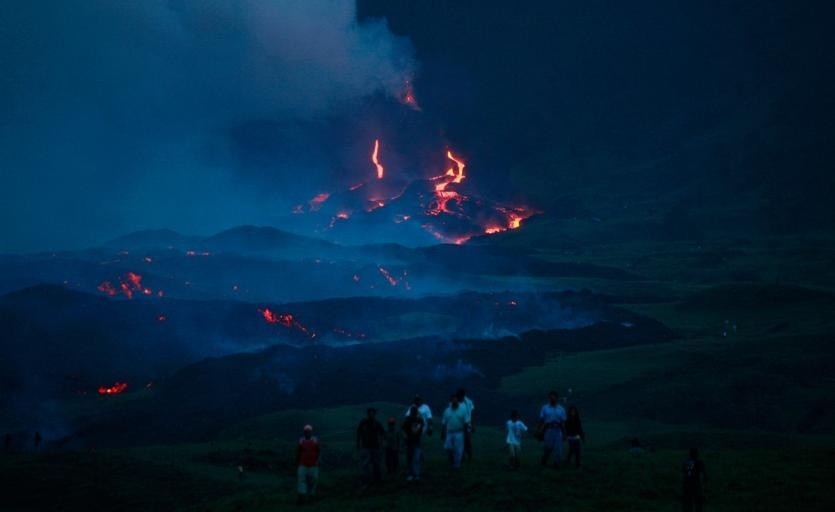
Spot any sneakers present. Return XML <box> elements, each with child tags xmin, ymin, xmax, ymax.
<box><xmin>406</xmin><ymin>475</ymin><xmax>414</xmax><ymax>481</ymax></box>
<box><xmin>414</xmin><ymin>475</ymin><xmax>421</xmax><ymax>481</ymax></box>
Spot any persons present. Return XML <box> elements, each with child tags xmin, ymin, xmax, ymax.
<box><xmin>678</xmin><ymin>447</ymin><xmax>711</xmax><ymax>512</ymax></box>
<box><xmin>294</xmin><ymin>385</ymin><xmax>586</xmax><ymax>503</ymax></box>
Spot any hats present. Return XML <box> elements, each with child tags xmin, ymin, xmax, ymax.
<box><xmin>387</xmin><ymin>417</ymin><xmax>396</xmax><ymax>424</ymax></box>
<box><xmin>303</xmin><ymin>424</ymin><xmax>312</xmax><ymax>432</ymax></box>
